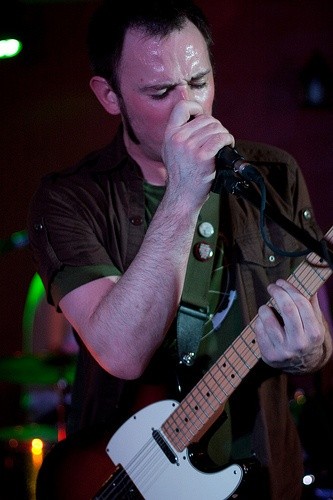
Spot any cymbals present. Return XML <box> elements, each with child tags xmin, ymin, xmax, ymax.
<box><xmin>0</xmin><ymin>353</ymin><xmax>81</xmax><ymax>389</ymax></box>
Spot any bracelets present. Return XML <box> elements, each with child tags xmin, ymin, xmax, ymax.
<box><xmin>313</xmin><ymin>340</ymin><xmax>327</xmax><ymax>371</ymax></box>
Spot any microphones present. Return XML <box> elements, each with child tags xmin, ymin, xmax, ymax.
<box><xmin>187</xmin><ymin>115</ymin><xmax>262</xmax><ymax>182</ymax></box>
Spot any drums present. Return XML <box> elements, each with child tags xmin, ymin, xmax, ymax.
<box><xmin>0</xmin><ymin>424</ymin><xmax>65</xmax><ymax>500</ymax></box>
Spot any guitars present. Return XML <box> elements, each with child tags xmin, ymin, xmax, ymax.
<box><xmin>34</xmin><ymin>226</ymin><xmax>333</xmax><ymax>499</ymax></box>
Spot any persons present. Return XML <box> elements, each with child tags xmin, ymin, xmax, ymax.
<box><xmin>31</xmin><ymin>0</ymin><xmax>333</xmax><ymax>500</ymax></box>
